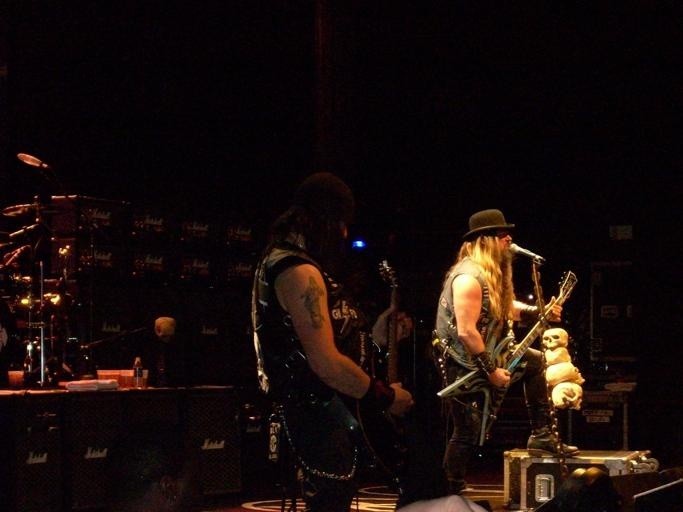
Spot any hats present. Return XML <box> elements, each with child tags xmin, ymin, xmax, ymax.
<box><xmin>463</xmin><ymin>208</ymin><xmax>515</xmax><ymax>239</ymax></box>
<box><xmin>297</xmin><ymin>173</ymin><xmax>354</xmax><ymax>219</ymax></box>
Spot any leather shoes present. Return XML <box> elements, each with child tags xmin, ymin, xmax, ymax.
<box><xmin>527</xmin><ymin>426</ymin><xmax>579</xmax><ymax>458</ymax></box>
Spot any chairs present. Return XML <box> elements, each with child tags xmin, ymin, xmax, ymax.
<box><xmin>96</xmin><ymin>367</ymin><xmax>149</xmax><ymax>388</ymax></box>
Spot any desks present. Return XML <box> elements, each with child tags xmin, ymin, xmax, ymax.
<box><xmin>1</xmin><ymin>385</ymin><xmax>242</xmax><ymax>511</ymax></box>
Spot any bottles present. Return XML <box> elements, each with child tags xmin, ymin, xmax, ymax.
<box><xmin>132</xmin><ymin>362</ymin><xmax>143</xmax><ymax>388</ymax></box>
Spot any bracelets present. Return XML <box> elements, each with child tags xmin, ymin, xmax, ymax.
<box><xmin>357</xmin><ymin>376</ymin><xmax>396</xmax><ymax>420</ymax></box>
<box><xmin>519</xmin><ymin>302</ymin><xmax>540</xmax><ymax>325</ymax></box>
<box><xmin>469</xmin><ymin>350</ymin><xmax>497</xmax><ymax>379</ymax></box>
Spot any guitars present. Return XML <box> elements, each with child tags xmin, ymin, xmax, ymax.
<box><xmin>437</xmin><ymin>270</ymin><xmax>579</xmax><ymax>446</ymax></box>
<box><xmin>355</xmin><ymin>260</ymin><xmax>411</xmax><ymax>471</ymax></box>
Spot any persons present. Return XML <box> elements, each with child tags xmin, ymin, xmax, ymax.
<box><xmin>431</xmin><ymin>209</ymin><xmax>582</xmax><ymax>490</ymax></box>
<box><xmin>394</xmin><ymin>494</ymin><xmax>489</xmax><ymax>512</ymax></box>
<box><xmin>250</xmin><ymin>169</ymin><xmax>418</xmax><ymax>511</ymax></box>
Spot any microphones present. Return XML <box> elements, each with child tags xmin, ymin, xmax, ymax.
<box><xmin>506</xmin><ymin>243</ymin><xmax>547</xmax><ymax>264</ymax></box>
